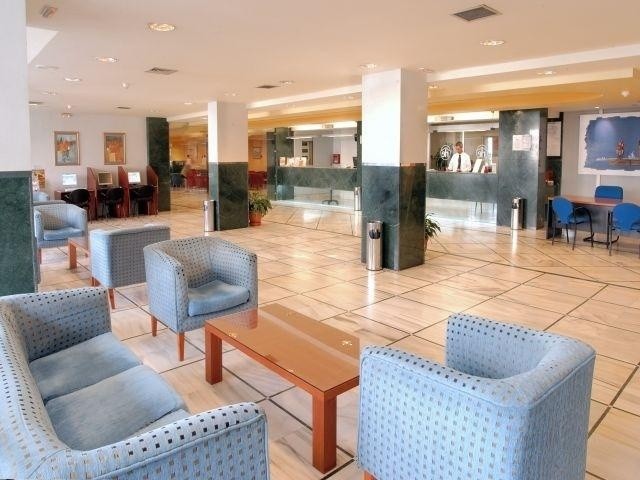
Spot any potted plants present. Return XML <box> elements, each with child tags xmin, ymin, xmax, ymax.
<box><xmin>250</xmin><ymin>190</ymin><xmax>272</xmax><ymax>227</ymax></box>
<box><xmin>425</xmin><ymin>211</ymin><xmax>442</xmax><ymax>252</ymax></box>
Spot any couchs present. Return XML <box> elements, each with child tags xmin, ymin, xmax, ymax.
<box><xmin>0</xmin><ymin>277</ymin><xmax>276</xmax><ymax>480</ymax></box>
<box><xmin>29</xmin><ymin>190</ymin><xmax>51</xmax><ymax>208</ymax></box>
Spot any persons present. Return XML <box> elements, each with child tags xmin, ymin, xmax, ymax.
<box><xmin>444</xmin><ymin>141</ymin><xmax>473</xmax><ymax>174</ymax></box>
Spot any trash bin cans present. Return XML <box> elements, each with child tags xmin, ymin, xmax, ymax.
<box><xmin>354</xmin><ymin>186</ymin><xmax>362</xmax><ymax>211</ymax></box>
<box><xmin>366</xmin><ymin>221</ymin><xmax>383</xmax><ymax>271</ymax></box>
<box><xmin>203</xmin><ymin>200</ymin><xmax>214</xmax><ymax>232</ymax></box>
<box><xmin>511</xmin><ymin>197</ymin><xmax>524</xmax><ymax>229</ymax></box>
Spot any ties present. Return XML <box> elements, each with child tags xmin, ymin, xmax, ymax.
<box><xmin>458</xmin><ymin>155</ymin><xmax>461</xmax><ymax>168</ymax></box>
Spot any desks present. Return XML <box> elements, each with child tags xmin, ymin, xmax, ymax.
<box><xmin>90</xmin><ymin>183</ymin><xmax>128</xmax><ymax>224</ymax></box>
<box><xmin>64</xmin><ymin>236</ymin><xmax>93</xmax><ymax>273</ymax></box>
<box><xmin>202</xmin><ymin>300</ymin><xmax>377</xmax><ymax>474</ymax></box>
<box><xmin>124</xmin><ymin>183</ymin><xmax>158</xmax><ymax>219</ymax></box>
<box><xmin>55</xmin><ymin>185</ymin><xmax>95</xmax><ymax>224</ymax></box>
<box><xmin>551</xmin><ymin>188</ymin><xmax>639</xmax><ymax>248</ymax></box>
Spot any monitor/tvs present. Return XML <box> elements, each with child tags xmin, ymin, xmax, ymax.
<box><xmin>97</xmin><ymin>172</ymin><xmax>113</xmax><ymax>185</ymax></box>
<box><xmin>127</xmin><ymin>171</ymin><xmax>141</xmax><ymax>184</ymax></box>
<box><xmin>62</xmin><ymin>174</ymin><xmax>77</xmax><ymax>186</ymax></box>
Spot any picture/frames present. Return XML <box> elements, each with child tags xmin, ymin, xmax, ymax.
<box><xmin>250</xmin><ymin>145</ymin><xmax>262</xmax><ymax>159</ymax></box>
<box><xmin>53</xmin><ymin>130</ymin><xmax>81</xmax><ymax>168</ymax></box>
<box><xmin>103</xmin><ymin>132</ymin><xmax>126</xmax><ymax>167</ymax></box>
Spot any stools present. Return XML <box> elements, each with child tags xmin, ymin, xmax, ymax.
<box><xmin>321</xmin><ymin>188</ymin><xmax>340</xmax><ymax>207</ymax></box>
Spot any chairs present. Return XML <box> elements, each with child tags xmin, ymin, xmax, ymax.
<box><xmin>474</xmin><ymin>202</ymin><xmax>495</xmax><ymax>215</ymax></box>
<box><xmin>139</xmin><ymin>233</ymin><xmax>259</xmax><ymax>360</ymax></box>
<box><xmin>82</xmin><ymin>217</ymin><xmax>173</xmax><ymax>309</ymax></box>
<box><xmin>172</xmin><ymin>176</ymin><xmax>185</xmax><ymax>190</ymax></box>
<box><xmin>102</xmin><ymin>185</ymin><xmax>125</xmax><ymax>221</ymax></box>
<box><xmin>67</xmin><ymin>188</ymin><xmax>89</xmax><ymax>220</ymax></box>
<box><xmin>248</xmin><ymin>169</ymin><xmax>267</xmax><ymax>189</ymax></box>
<box><xmin>132</xmin><ymin>185</ymin><xmax>157</xmax><ymax>217</ymax></box>
<box><xmin>603</xmin><ymin>198</ymin><xmax>639</xmax><ymax>257</ymax></box>
<box><xmin>186</xmin><ymin>168</ymin><xmax>209</xmax><ymax>192</ymax></box>
<box><xmin>586</xmin><ymin>182</ymin><xmax>625</xmax><ymax>246</ymax></box>
<box><xmin>27</xmin><ymin>202</ymin><xmax>86</xmax><ymax>271</ymax></box>
<box><xmin>544</xmin><ymin>194</ymin><xmax>594</xmax><ymax>247</ymax></box>
<box><xmin>356</xmin><ymin>307</ymin><xmax>595</xmax><ymax>480</ymax></box>
